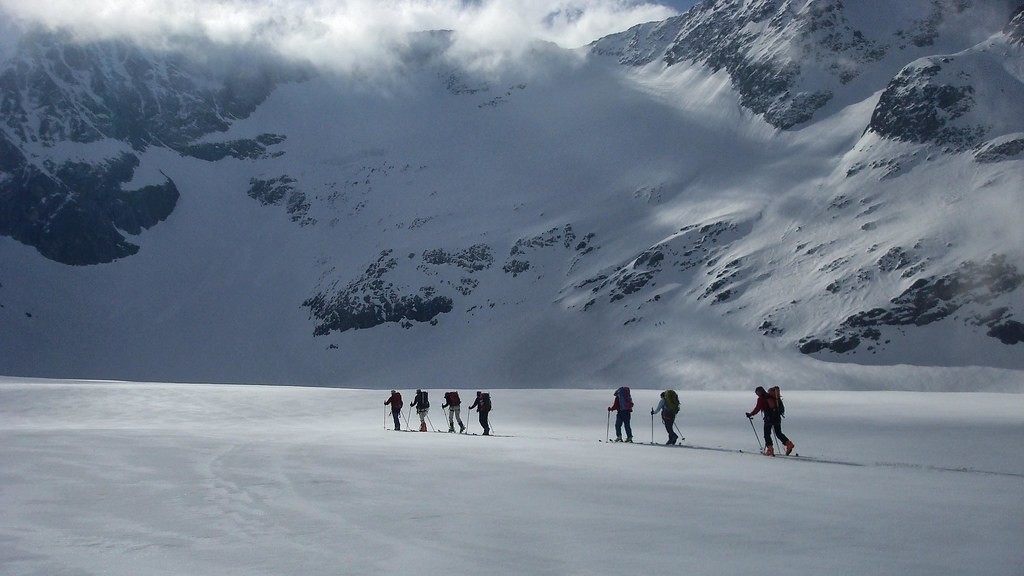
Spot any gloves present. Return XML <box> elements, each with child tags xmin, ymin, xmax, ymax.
<box><xmin>746</xmin><ymin>413</ymin><xmax>751</xmax><ymax>418</ymax></box>
<box><xmin>651</xmin><ymin>411</ymin><xmax>654</xmax><ymax>415</ymax></box>
<box><xmin>608</xmin><ymin>408</ymin><xmax>611</xmax><ymax>411</ymax></box>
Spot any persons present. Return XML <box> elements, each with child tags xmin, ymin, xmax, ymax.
<box><xmin>384</xmin><ymin>390</ymin><xmax>403</xmax><ymax>431</ymax></box>
<box><xmin>607</xmin><ymin>389</ymin><xmax>634</xmax><ymax>443</ymax></box>
<box><xmin>410</xmin><ymin>389</ymin><xmax>430</xmax><ymax>432</ymax></box>
<box><xmin>468</xmin><ymin>391</ymin><xmax>490</xmax><ymax>435</ymax></box>
<box><xmin>442</xmin><ymin>392</ymin><xmax>466</xmax><ymax>434</ymax></box>
<box><xmin>651</xmin><ymin>391</ymin><xmax>679</xmax><ymax>446</ymax></box>
<box><xmin>745</xmin><ymin>386</ymin><xmax>795</xmax><ymax>457</ymax></box>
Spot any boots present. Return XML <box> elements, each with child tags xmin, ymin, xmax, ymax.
<box><xmin>784</xmin><ymin>440</ymin><xmax>794</xmax><ymax>455</ymax></box>
<box><xmin>449</xmin><ymin>423</ymin><xmax>454</xmax><ymax>432</ymax></box>
<box><xmin>765</xmin><ymin>445</ymin><xmax>774</xmax><ymax>456</ymax></box>
<box><xmin>666</xmin><ymin>432</ymin><xmax>678</xmax><ymax>445</ymax></box>
<box><xmin>420</xmin><ymin>423</ymin><xmax>427</xmax><ymax>432</ymax></box>
<box><xmin>458</xmin><ymin>421</ymin><xmax>465</xmax><ymax>433</ymax></box>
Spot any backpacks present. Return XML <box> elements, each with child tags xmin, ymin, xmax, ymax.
<box><xmin>664</xmin><ymin>390</ymin><xmax>680</xmax><ymax>415</ymax></box>
<box><xmin>449</xmin><ymin>392</ymin><xmax>461</xmax><ymax>406</ymax></box>
<box><xmin>393</xmin><ymin>393</ymin><xmax>403</xmax><ymax>410</ymax></box>
<box><xmin>616</xmin><ymin>387</ymin><xmax>634</xmax><ymax>412</ymax></box>
<box><xmin>759</xmin><ymin>386</ymin><xmax>782</xmax><ymax>415</ymax></box>
<box><xmin>418</xmin><ymin>392</ymin><xmax>429</xmax><ymax>409</ymax></box>
<box><xmin>480</xmin><ymin>393</ymin><xmax>491</xmax><ymax>413</ymax></box>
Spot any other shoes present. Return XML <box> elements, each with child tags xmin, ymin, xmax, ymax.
<box><xmin>625</xmin><ymin>436</ymin><xmax>632</xmax><ymax>443</ymax></box>
<box><xmin>615</xmin><ymin>435</ymin><xmax>622</xmax><ymax>442</ymax></box>
